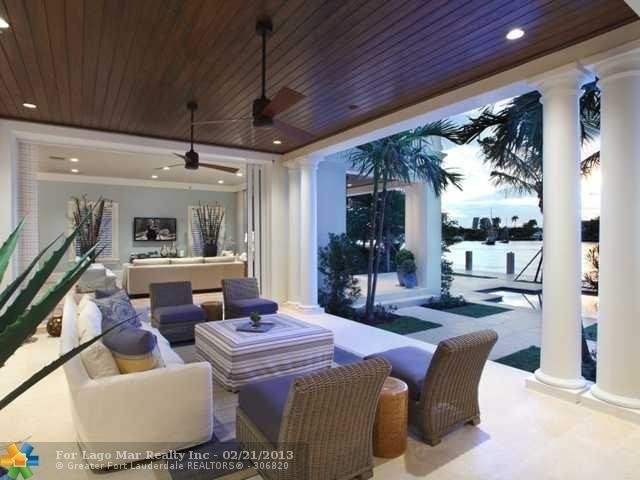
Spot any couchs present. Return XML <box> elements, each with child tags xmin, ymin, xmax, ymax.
<box><xmin>121</xmin><ymin>255</ymin><xmax>246</xmax><ymax>298</ymax></box>
<box><xmin>58</xmin><ymin>292</ymin><xmax>215</xmax><ymax>471</ymax></box>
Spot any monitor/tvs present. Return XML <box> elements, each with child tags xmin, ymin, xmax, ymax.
<box><xmin>134</xmin><ymin>218</ymin><xmax>176</xmax><ymax>241</ymax></box>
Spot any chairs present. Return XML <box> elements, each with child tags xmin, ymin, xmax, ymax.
<box><xmin>74</xmin><ymin>255</ymin><xmax>116</xmax><ymax>292</ymax></box>
<box><xmin>149</xmin><ymin>277</ymin><xmax>278</xmax><ymax>343</ymax></box>
<box><xmin>233</xmin><ymin>328</ymin><xmax>500</xmax><ymax>480</ymax></box>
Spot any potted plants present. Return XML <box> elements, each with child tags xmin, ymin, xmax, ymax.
<box><xmin>249</xmin><ymin>310</ymin><xmax>263</xmax><ymax>328</ymax></box>
<box><xmin>197</xmin><ymin>198</ymin><xmax>225</xmax><ymax>257</ymax></box>
<box><xmin>395</xmin><ymin>248</ymin><xmax>417</xmax><ymax>290</ymax></box>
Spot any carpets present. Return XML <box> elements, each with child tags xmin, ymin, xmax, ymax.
<box><xmin>149</xmin><ymin>338</ymin><xmax>365</xmax><ymax>480</ymax></box>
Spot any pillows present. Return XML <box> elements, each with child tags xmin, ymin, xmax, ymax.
<box><xmin>77</xmin><ymin>288</ymin><xmax>164</xmax><ymax>379</ymax></box>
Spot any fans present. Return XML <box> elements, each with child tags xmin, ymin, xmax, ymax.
<box><xmin>151</xmin><ymin>14</ymin><xmax>316</xmax><ymax>173</ymax></box>
